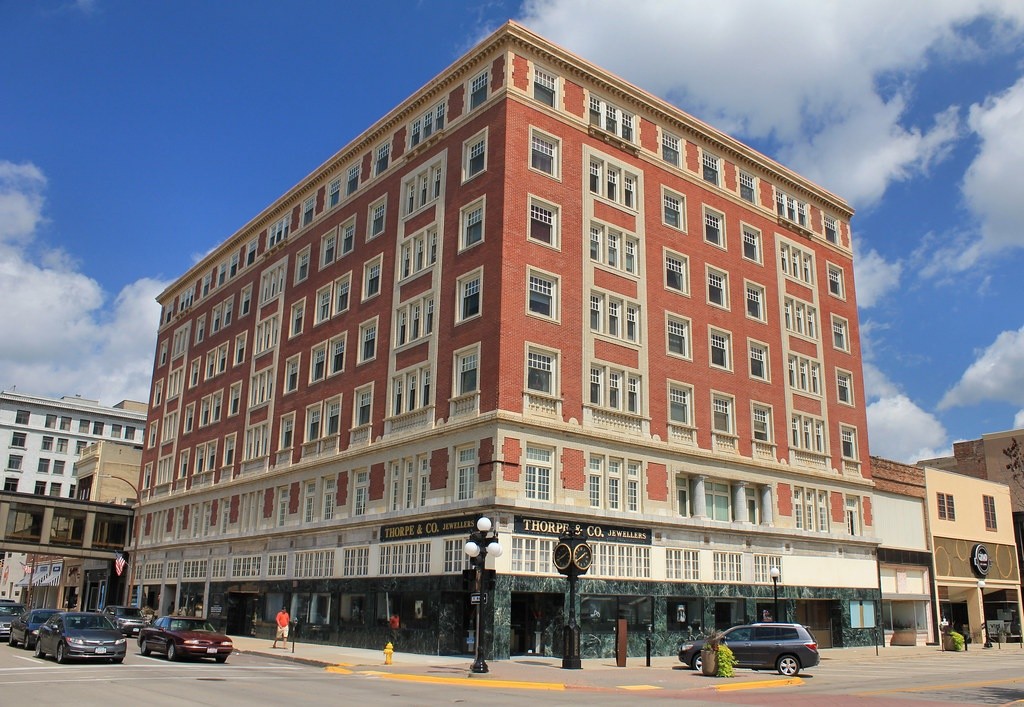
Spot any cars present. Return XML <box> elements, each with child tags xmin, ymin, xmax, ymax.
<box><xmin>35</xmin><ymin>611</ymin><xmax>128</xmax><ymax>664</ymax></box>
<box><xmin>0</xmin><ymin>598</ymin><xmax>15</xmax><ymax>605</ymax></box>
<box><xmin>136</xmin><ymin>615</ymin><xmax>234</xmax><ymax>663</ymax></box>
<box><xmin>0</xmin><ymin>602</ymin><xmax>30</xmax><ymax>639</ymax></box>
<box><xmin>99</xmin><ymin>605</ymin><xmax>150</xmax><ymax>638</ymax></box>
<box><xmin>8</xmin><ymin>609</ymin><xmax>67</xmax><ymax>650</ymax></box>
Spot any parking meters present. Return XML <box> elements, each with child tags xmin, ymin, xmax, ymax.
<box><xmin>292</xmin><ymin>617</ymin><xmax>299</xmax><ymax>654</ymax></box>
<box><xmin>644</xmin><ymin>623</ymin><xmax>655</xmax><ymax>669</ymax></box>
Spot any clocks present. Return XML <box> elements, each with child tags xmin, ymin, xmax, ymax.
<box><xmin>573</xmin><ymin>544</ymin><xmax>593</xmax><ymax>570</ymax></box>
<box><xmin>552</xmin><ymin>543</ymin><xmax>571</xmax><ymax>570</ymax></box>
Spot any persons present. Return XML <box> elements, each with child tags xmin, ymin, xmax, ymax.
<box><xmin>272</xmin><ymin>606</ymin><xmax>290</xmax><ymax>649</ymax></box>
<box><xmin>390</xmin><ymin>610</ymin><xmax>400</xmax><ymax>629</ymax></box>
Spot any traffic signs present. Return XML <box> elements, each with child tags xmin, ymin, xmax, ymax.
<box><xmin>470</xmin><ymin>593</ymin><xmax>488</xmax><ymax>605</ymax></box>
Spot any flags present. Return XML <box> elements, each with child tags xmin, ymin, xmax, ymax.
<box><xmin>115</xmin><ymin>552</ymin><xmax>125</xmax><ymax>576</ymax></box>
<box><xmin>20</xmin><ymin>563</ymin><xmax>31</xmax><ymax>573</ymax></box>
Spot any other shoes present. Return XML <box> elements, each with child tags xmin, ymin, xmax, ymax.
<box><xmin>273</xmin><ymin>645</ymin><xmax>277</xmax><ymax>647</ymax></box>
<box><xmin>284</xmin><ymin>647</ymin><xmax>288</xmax><ymax>649</ymax></box>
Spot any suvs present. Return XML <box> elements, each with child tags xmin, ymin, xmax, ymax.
<box><xmin>679</xmin><ymin>621</ymin><xmax>822</xmax><ymax>677</ymax></box>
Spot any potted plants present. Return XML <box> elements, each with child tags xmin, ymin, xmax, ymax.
<box><xmin>943</xmin><ymin>626</ymin><xmax>964</xmax><ymax>651</ymax></box>
<box><xmin>700</xmin><ymin>639</ymin><xmax>739</xmax><ymax>678</ymax></box>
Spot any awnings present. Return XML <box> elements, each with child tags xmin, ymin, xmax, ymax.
<box><xmin>15</xmin><ymin>572</ymin><xmax>60</xmax><ymax>586</ymax></box>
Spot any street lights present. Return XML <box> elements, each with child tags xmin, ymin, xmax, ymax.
<box><xmin>769</xmin><ymin>567</ymin><xmax>780</xmax><ymax>624</ymax></box>
<box><xmin>978</xmin><ymin>581</ymin><xmax>992</xmax><ymax>649</ymax></box>
<box><xmin>462</xmin><ymin>516</ymin><xmax>504</xmax><ymax>672</ymax></box>
<box><xmin>97</xmin><ymin>474</ymin><xmax>142</xmax><ymax>604</ymax></box>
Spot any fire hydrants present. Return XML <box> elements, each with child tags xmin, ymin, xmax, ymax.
<box><xmin>383</xmin><ymin>641</ymin><xmax>395</xmax><ymax>666</ymax></box>
